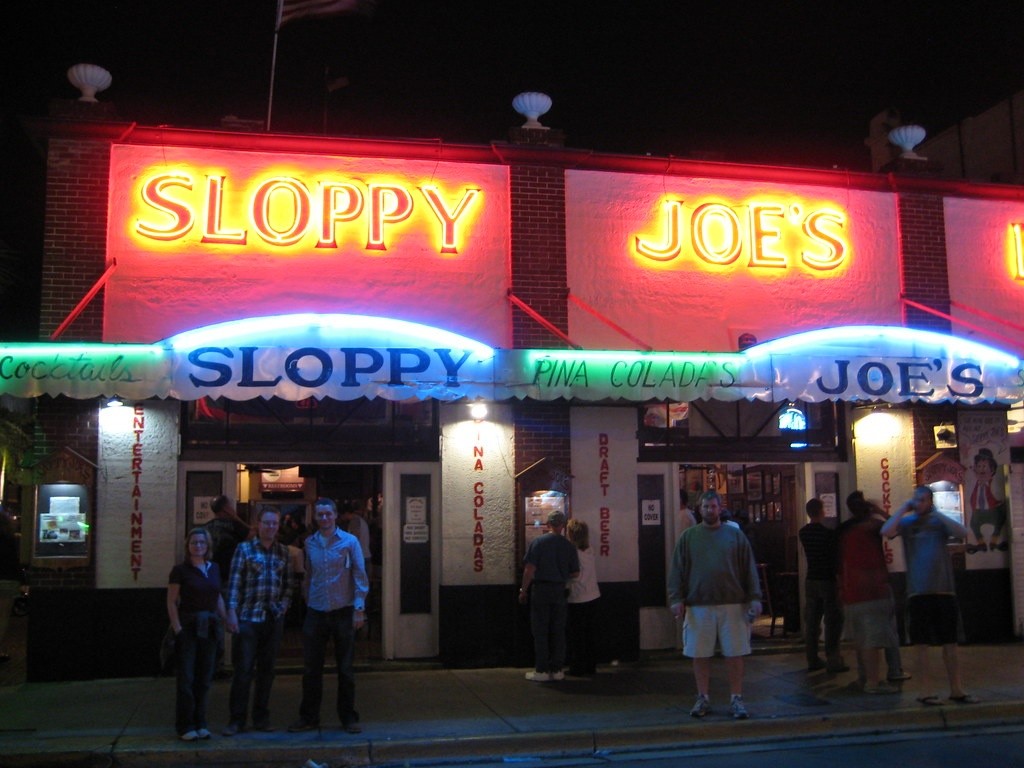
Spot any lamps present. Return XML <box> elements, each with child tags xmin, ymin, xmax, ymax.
<box><xmin>887</xmin><ymin>125</ymin><xmax>928</xmax><ymax>159</ymax></box>
<box><xmin>513</xmin><ymin>92</ymin><xmax>552</xmax><ymax>130</ymax></box>
<box><xmin>67</xmin><ymin>64</ymin><xmax>112</xmax><ymax>103</ymax></box>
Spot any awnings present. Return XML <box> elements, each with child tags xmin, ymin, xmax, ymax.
<box><xmin>0</xmin><ymin>314</ymin><xmax>1024</xmax><ymax>402</ymax></box>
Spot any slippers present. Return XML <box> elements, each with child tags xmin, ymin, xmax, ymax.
<box><xmin>916</xmin><ymin>695</ymin><xmax>944</xmax><ymax>706</ymax></box>
<box><xmin>949</xmin><ymin>695</ymin><xmax>976</xmax><ymax>704</ymax></box>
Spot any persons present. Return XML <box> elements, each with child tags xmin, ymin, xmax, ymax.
<box><xmin>680</xmin><ymin>489</ymin><xmax>696</xmax><ymax>532</ymax></box>
<box><xmin>565</xmin><ymin>519</ymin><xmax>601</xmax><ymax>677</ymax></box>
<box><xmin>167</xmin><ymin>527</ymin><xmax>240</xmax><ymax>741</ymax></box>
<box><xmin>338</xmin><ymin>505</ymin><xmax>371</xmax><ymax>575</ymax></box>
<box><xmin>879</xmin><ymin>486</ymin><xmax>982</xmax><ymax>706</ymax></box>
<box><xmin>835</xmin><ymin>490</ymin><xmax>912</xmax><ymax>694</ymax></box>
<box><xmin>200</xmin><ymin>494</ymin><xmax>252</xmax><ymax>679</ymax></box>
<box><xmin>798</xmin><ymin>499</ymin><xmax>850</xmax><ymax>673</ymax></box>
<box><xmin>222</xmin><ymin>506</ymin><xmax>299</xmax><ymax>736</ymax></box>
<box><xmin>518</xmin><ymin>511</ymin><xmax>580</xmax><ymax>681</ymax></box>
<box><xmin>667</xmin><ymin>491</ymin><xmax>763</xmax><ymax>719</ymax></box>
<box><xmin>274</xmin><ymin>512</ymin><xmax>306</xmax><ymax>573</ymax></box>
<box><xmin>288</xmin><ymin>499</ymin><xmax>370</xmax><ymax>733</ymax></box>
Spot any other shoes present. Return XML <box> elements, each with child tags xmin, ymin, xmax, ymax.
<box><xmin>178</xmin><ymin>730</ymin><xmax>198</xmax><ymax>740</ymax></box>
<box><xmin>287</xmin><ymin>719</ymin><xmax>320</xmax><ymax>731</ymax></box>
<box><xmin>551</xmin><ymin>671</ymin><xmax>564</xmax><ymax>680</ymax></box>
<box><xmin>886</xmin><ymin>670</ymin><xmax>912</xmax><ymax>680</ymax></box>
<box><xmin>196</xmin><ymin>728</ymin><xmax>211</xmax><ymax>738</ymax></box>
<box><xmin>826</xmin><ymin>662</ymin><xmax>849</xmax><ymax>672</ymax></box>
<box><xmin>525</xmin><ymin>671</ymin><xmax>550</xmax><ymax>681</ymax></box>
<box><xmin>857</xmin><ymin>679</ymin><xmax>879</xmax><ymax>693</ymax></box>
<box><xmin>343</xmin><ymin>722</ymin><xmax>361</xmax><ymax>732</ymax></box>
<box><xmin>254</xmin><ymin>722</ymin><xmax>274</xmax><ymax>731</ymax></box>
<box><xmin>808</xmin><ymin>660</ymin><xmax>826</xmax><ymax>670</ymax></box>
<box><xmin>221</xmin><ymin>724</ymin><xmax>242</xmax><ymax>735</ymax></box>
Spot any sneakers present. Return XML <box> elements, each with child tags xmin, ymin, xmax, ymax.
<box><xmin>728</xmin><ymin>696</ymin><xmax>749</xmax><ymax>718</ymax></box>
<box><xmin>689</xmin><ymin>696</ymin><xmax>711</xmax><ymax>716</ymax></box>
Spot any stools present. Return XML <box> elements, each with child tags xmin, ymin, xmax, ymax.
<box><xmin>770</xmin><ymin>572</ymin><xmax>799</xmax><ymax>637</ymax></box>
<box><xmin>756</xmin><ymin>563</ymin><xmax>772</xmax><ymax>619</ymax></box>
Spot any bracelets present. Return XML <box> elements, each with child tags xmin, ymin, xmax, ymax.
<box><xmin>520</xmin><ymin>588</ymin><xmax>526</xmax><ymax>593</ymax></box>
